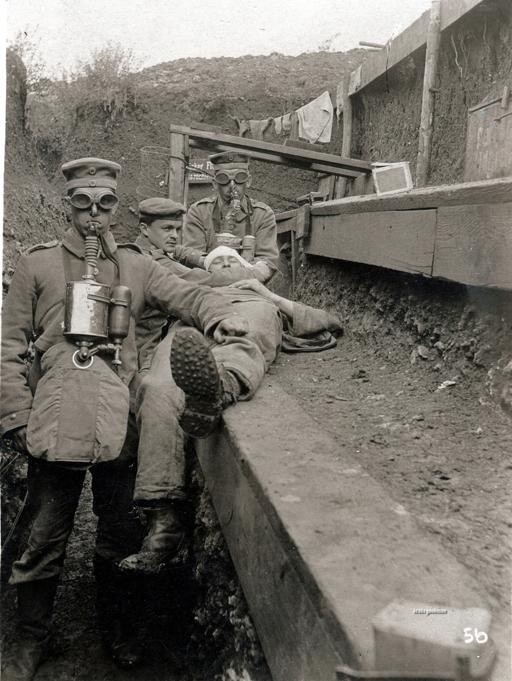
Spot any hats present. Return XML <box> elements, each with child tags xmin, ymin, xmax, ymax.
<box><xmin>61</xmin><ymin>157</ymin><xmax>122</xmax><ymax>190</ymax></box>
<box><xmin>139</xmin><ymin>198</ymin><xmax>188</xmax><ymax>222</ymax></box>
<box><xmin>207</xmin><ymin>149</ymin><xmax>251</xmax><ymax>171</ymax></box>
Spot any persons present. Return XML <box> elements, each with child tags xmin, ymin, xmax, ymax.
<box><xmin>4</xmin><ymin>157</ymin><xmax>250</xmax><ymax>668</ymax></box>
<box><xmin>183</xmin><ymin>148</ymin><xmax>283</xmax><ymax>285</ymax></box>
<box><xmin>117</xmin><ymin>246</ymin><xmax>342</xmax><ymax>591</ymax></box>
<box><xmin>119</xmin><ymin>196</ymin><xmax>273</xmax><ymax>294</ymax></box>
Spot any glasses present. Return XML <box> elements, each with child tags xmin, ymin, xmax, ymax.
<box><xmin>211</xmin><ymin>172</ymin><xmax>251</xmax><ymax>186</ymax></box>
<box><xmin>65</xmin><ymin>189</ymin><xmax>119</xmax><ymax>212</ymax></box>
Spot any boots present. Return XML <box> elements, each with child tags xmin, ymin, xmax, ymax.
<box><xmin>0</xmin><ymin>576</ymin><xmax>61</xmax><ymax>681</ymax></box>
<box><xmin>115</xmin><ymin>503</ymin><xmax>196</xmax><ymax>578</ymax></box>
<box><xmin>92</xmin><ymin>554</ymin><xmax>146</xmax><ymax>671</ymax></box>
<box><xmin>170</xmin><ymin>327</ymin><xmax>242</xmax><ymax>439</ymax></box>
